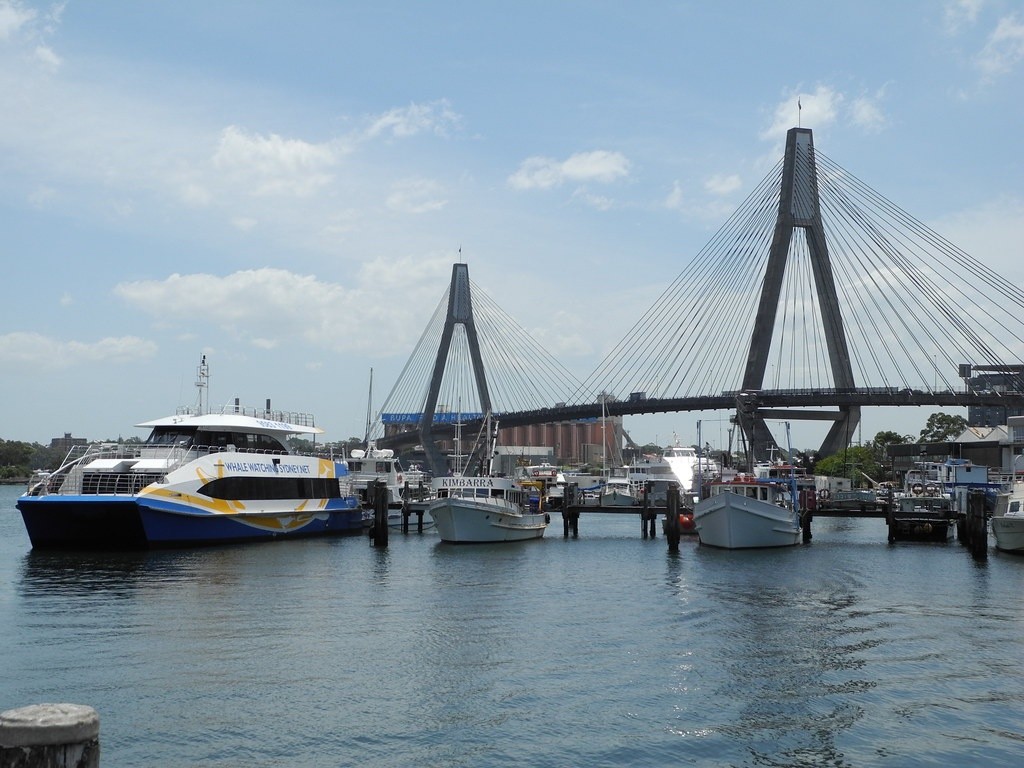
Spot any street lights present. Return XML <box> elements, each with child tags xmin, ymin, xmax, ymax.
<box><xmin>920</xmin><ymin>451</ymin><xmax>927</xmax><ymax>496</ymax></box>
<box><xmin>934</xmin><ymin>355</ymin><xmax>936</xmax><ymax>394</ymax></box>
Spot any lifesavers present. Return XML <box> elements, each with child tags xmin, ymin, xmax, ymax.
<box><xmin>819</xmin><ymin>488</ymin><xmax>830</xmax><ymax>499</ymax></box>
<box><xmin>896</xmin><ymin>470</ymin><xmax>901</xmax><ymax>475</ymax></box>
<box><xmin>1015</xmin><ymin>469</ymin><xmax>1024</xmax><ymax>474</ymax></box>
<box><xmin>914</xmin><ymin>526</ymin><xmax>922</xmax><ymax>535</ymax></box>
<box><xmin>552</xmin><ymin>470</ymin><xmax>557</xmax><ymax>476</ymax></box>
<box><xmin>924</xmin><ymin>523</ymin><xmax>932</xmax><ymax>533</ymax></box>
<box><xmin>545</xmin><ymin>513</ymin><xmax>550</xmax><ymax>523</ymax></box>
<box><xmin>734</xmin><ymin>477</ymin><xmax>755</xmax><ymax>482</ymax></box>
<box><xmin>737</xmin><ymin>472</ymin><xmax>753</xmax><ymax>477</ymax></box>
<box><xmin>913</xmin><ymin>483</ymin><xmax>923</xmax><ymax>494</ymax></box>
<box><xmin>397</xmin><ymin>474</ymin><xmax>403</xmax><ymax>483</ymax></box>
<box><xmin>926</xmin><ymin>483</ymin><xmax>937</xmax><ymax>494</ymax></box>
<box><xmin>533</xmin><ymin>471</ymin><xmax>539</xmax><ymax>476</ymax></box>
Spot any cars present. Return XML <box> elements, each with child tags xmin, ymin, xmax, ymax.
<box><xmin>874</xmin><ymin>482</ymin><xmax>894</xmax><ymax>497</ymax></box>
<box><xmin>899</xmin><ymin>389</ymin><xmax>912</xmax><ymax>394</ymax></box>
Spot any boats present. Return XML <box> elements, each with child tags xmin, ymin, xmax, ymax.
<box><xmin>830</xmin><ymin>488</ymin><xmax>877</xmax><ymax>510</ymax></box>
<box><xmin>16</xmin><ymin>354</ymin><xmax>361</xmax><ymax>549</ymax></box>
<box><xmin>426</xmin><ymin>396</ymin><xmax>551</xmax><ymax>542</ymax></box>
<box><xmin>894</xmin><ymin>496</ymin><xmax>957</xmax><ymax>539</ymax></box>
<box><xmin>600</xmin><ymin>479</ymin><xmax>640</xmax><ymax>506</ymax></box>
<box><xmin>692</xmin><ymin>472</ymin><xmax>813</xmax><ymax>548</ymax></box>
<box><xmin>991</xmin><ymin>479</ymin><xmax>1024</xmax><ymax>552</ymax></box>
<box><xmin>609</xmin><ymin>447</ymin><xmax>720</xmax><ymax>491</ymax></box>
<box><xmin>577</xmin><ymin>484</ymin><xmax>604</xmax><ymax>507</ymax></box>
<box><xmin>345</xmin><ymin>449</ymin><xmax>428</xmax><ymax>497</ymax></box>
<box><xmin>662</xmin><ymin>492</ymin><xmax>698</xmax><ymax>535</ymax></box>
